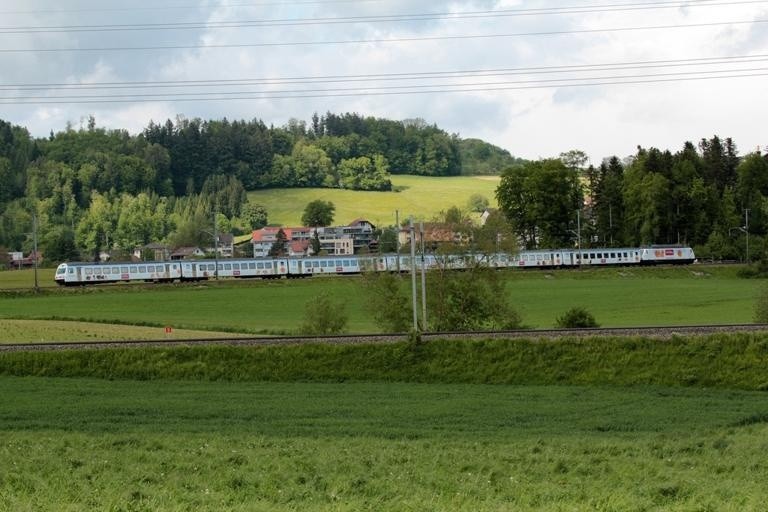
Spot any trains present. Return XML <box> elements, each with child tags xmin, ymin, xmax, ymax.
<box><xmin>52</xmin><ymin>243</ymin><xmax>698</xmax><ymax>288</ymax></box>
